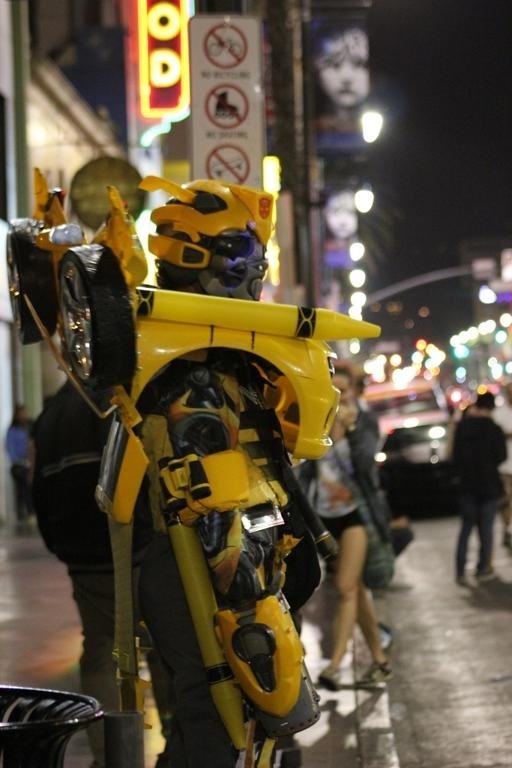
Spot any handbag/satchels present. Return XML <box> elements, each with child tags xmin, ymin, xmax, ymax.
<box><xmin>362</xmin><ymin>522</ymin><xmax>396</xmax><ymax>589</ymax></box>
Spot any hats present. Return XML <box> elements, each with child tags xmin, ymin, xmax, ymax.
<box><xmin>474</xmin><ymin>391</ymin><xmax>495</xmax><ymax>409</ymax></box>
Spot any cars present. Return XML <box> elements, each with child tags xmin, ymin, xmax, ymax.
<box><xmin>367</xmin><ymin>382</ymin><xmax>466</xmax><ymax>517</ymax></box>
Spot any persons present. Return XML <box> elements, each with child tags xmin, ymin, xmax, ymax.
<box><xmin>8</xmin><ymin>168</ymin><xmax>381</xmax><ymax>767</ymax></box>
<box><xmin>314</xmin><ymin>18</ymin><xmax>371</xmax><ymax>139</ymax></box>
<box><xmin>320</xmin><ymin>175</ymin><xmax>359</xmax><ymax>252</ymax></box>
<box><xmin>330</xmin><ymin>384</ymin><xmax>512</xmax><ymax>588</ymax></box>
<box><xmin>25</xmin><ymin>372</ymin><xmax>178</xmax><ymax>768</ymax></box>
<box><xmin>294</xmin><ymin>356</ymin><xmax>394</xmax><ymax>690</ymax></box>
<box><xmin>6</xmin><ymin>405</ymin><xmax>38</xmax><ymax>527</ymax></box>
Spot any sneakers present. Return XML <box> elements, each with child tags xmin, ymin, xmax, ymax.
<box><xmin>321</xmin><ymin>659</ymin><xmax>392</xmax><ymax>690</ymax></box>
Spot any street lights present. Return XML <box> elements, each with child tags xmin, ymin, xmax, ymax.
<box><xmin>297</xmin><ymin>86</ymin><xmax>384</xmax><ymax>391</ymax></box>
